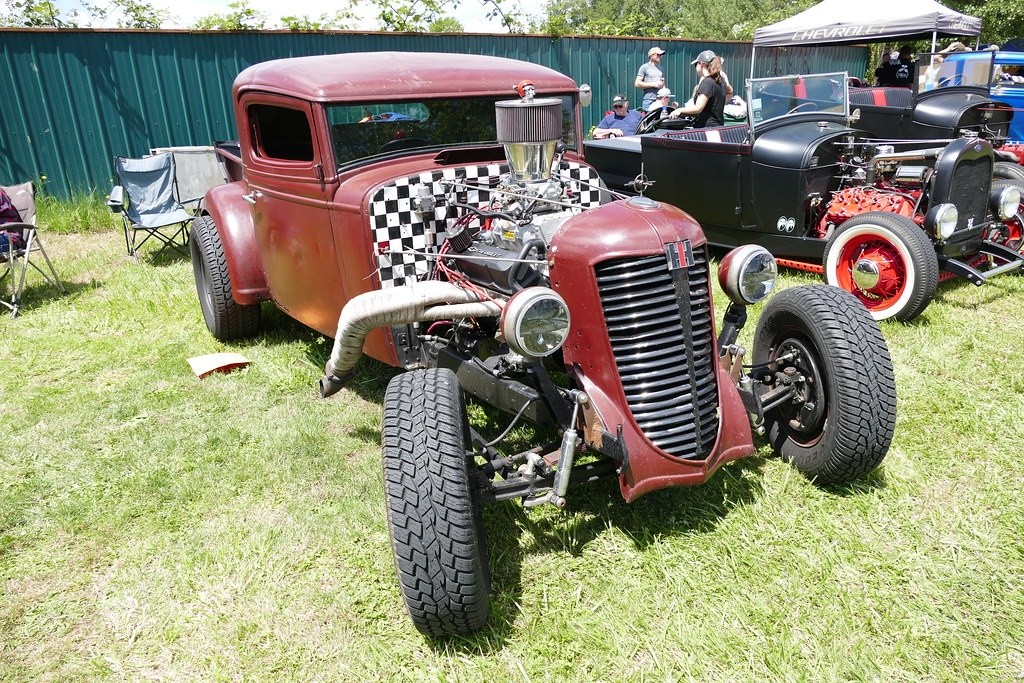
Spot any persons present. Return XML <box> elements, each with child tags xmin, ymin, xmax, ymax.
<box><xmin>0</xmin><ymin>187</ymin><xmax>26</xmax><ymax>253</ymax></box>
<box><xmin>649</xmin><ymin>88</ymin><xmax>678</xmax><ymax>113</ymax></box>
<box><xmin>635</xmin><ymin>47</ymin><xmax>665</xmax><ymax>110</ymax></box>
<box><xmin>593</xmin><ymin>95</ymin><xmax>643</xmax><ymax>137</ymax></box>
<box><xmin>669</xmin><ymin>50</ymin><xmax>726</xmax><ymax>129</ymax></box>
<box><xmin>875</xmin><ymin>42</ymin><xmax>1000</xmax><ymax>89</ymax></box>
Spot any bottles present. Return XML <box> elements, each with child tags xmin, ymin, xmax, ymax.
<box><xmin>660</xmin><ymin>106</ymin><xmax>669</xmax><ymax>122</ymax></box>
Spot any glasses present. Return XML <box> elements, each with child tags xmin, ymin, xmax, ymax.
<box><xmin>614</xmin><ymin>104</ymin><xmax>623</xmax><ymax>108</ymax></box>
<box><xmin>695</xmin><ymin>62</ymin><xmax>705</xmax><ymax>66</ymax></box>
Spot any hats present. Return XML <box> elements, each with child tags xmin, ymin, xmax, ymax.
<box><xmin>691</xmin><ymin>49</ymin><xmax>715</xmax><ymax>64</ymax></box>
<box><xmin>657</xmin><ymin>88</ymin><xmax>675</xmax><ymax>97</ymax></box>
<box><xmin>612</xmin><ymin>94</ymin><xmax>626</xmax><ymax>106</ymax></box>
<box><xmin>648</xmin><ymin>46</ymin><xmax>665</xmax><ymax>56</ymax></box>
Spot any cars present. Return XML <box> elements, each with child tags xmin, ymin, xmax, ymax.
<box><xmin>578</xmin><ymin>48</ymin><xmax>1024</xmax><ymax>329</ymax></box>
<box><xmin>187</xmin><ymin>49</ymin><xmax>900</xmax><ymax>640</ymax></box>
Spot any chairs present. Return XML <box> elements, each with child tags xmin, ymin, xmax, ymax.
<box><xmin>0</xmin><ymin>181</ymin><xmax>66</xmax><ymax>317</ymax></box>
<box><xmin>108</xmin><ymin>151</ymin><xmax>205</xmax><ymax>268</ymax></box>
<box><xmin>594</xmin><ymin>110</ymin><xmax>646</xmax><ymax>141</ymax></box>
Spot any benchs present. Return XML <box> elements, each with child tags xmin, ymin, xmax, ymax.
<box><xmin>662</xmin><ymin>126</ymin><xmax>751</xmax><ymax>143</ymax></box>
<box><xmin>260</xmin><ymin>120</ymin><xmax>460</xmax><ymax>163</ymax></box>
<box><xmin>836</xmin><ymin>85</ymin><xmax>914</xmax><ymax>109</ymax></box>
<box><xmin>760</xmin><ymin>77</ymin><xmax>834</xmax><ymax>100</ymax></box>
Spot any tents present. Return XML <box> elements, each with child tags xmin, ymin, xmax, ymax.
<box><xmin>749</xmin><ymin>1</ymin><xmax>982</xmax><ymax>122</ymax></box>
